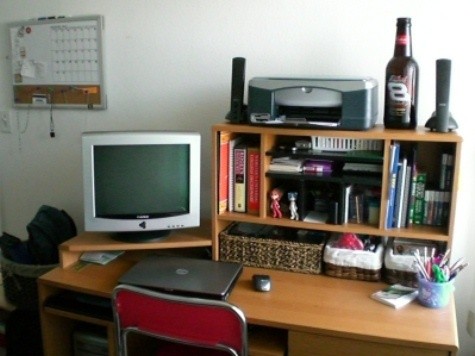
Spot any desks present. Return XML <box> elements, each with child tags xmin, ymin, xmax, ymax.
<box><xmin>37</xmin><ymin>250</ymin><xmax>423</xmax><ymax>355</ymax></box>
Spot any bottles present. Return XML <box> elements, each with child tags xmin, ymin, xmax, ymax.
<box><xmin>384</xmin><ymin>17</ymin><xmax>418</xmax><ymax>130</ymax></box>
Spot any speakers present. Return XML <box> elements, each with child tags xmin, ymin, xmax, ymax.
<box><xmin>224</xmin><ymin>57</ymin><xmax>248</xmax><ymax>124</ymax></box>
<box><xmin>425</xmin><ymin>58</ymin><xmax>456</xmax><ymax>132</ymax></box>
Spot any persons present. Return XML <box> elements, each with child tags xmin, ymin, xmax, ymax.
<box><xmin>287</xmin><ymin>191</ymin><xmax>300</xmax><ymax>221</ymax></box>
<box><xmin>269</xmin><ymin>187</ymin><xmax>283</xmax><ymax>218</ymax></box>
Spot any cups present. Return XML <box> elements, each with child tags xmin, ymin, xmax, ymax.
<box><xmin>416</xmin><ymin>275</ymin><xmax>456</xmax><ymax>306</ymax></box>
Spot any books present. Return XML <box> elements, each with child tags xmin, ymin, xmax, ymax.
<box><xmin>267</xmin><ymin>157</ymin><xmax>300</xmax><ymax>174</ymax></box>
<box><xmin>303</xmin><ymin>210</ymin><xmax>329</xmax><ymax>224</ymax></box>
<box><xmin>383</xmin><ymin>142</ymin><xmax>455</xmax><ymax>228</ymax></box>
<box><xmin>342</xmin><ymin>163</ymin><xmax>382</xmax><ymax>173</ymax></box>
<box><xmin>368</xmin><ymin>282</ymin><xmax>420</xmax><ymax>310</ymax></box>
<box><xmin>216</xmin><ymin>139</ymin><xmax>261</xmax><ymax>216</ymax></box>
<box><xmin>333</xmin><ymin>180</ymin><xmax>364</xmax><ymax>225</ymax></box>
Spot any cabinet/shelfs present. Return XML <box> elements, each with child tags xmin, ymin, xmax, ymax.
<box><xmin>211</xmin><ymin>123</ymin><xmax>463</xmax><ymax>287</ymax></box>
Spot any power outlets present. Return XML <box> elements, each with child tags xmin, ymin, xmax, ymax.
<box><xmin>0</xmin><ymin>110</ymin><xmax>12</xmax><ymax>134</ymax></box>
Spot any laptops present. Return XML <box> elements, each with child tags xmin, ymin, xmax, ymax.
<box><xmin>117</xmin><ymin>255</ymin><xmax>244</xmax><ymax>302</ymax></box>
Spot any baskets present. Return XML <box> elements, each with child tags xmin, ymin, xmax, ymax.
<box><xmin>1</xmin><ymin>247</ymin><xmax>58</xmax><ymax>279</ymax></box>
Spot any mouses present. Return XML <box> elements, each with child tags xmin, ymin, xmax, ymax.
<box><xmin>254</xmin><ymin>274</ymin><xmax>271</xmax><ymax>292</ymax></box>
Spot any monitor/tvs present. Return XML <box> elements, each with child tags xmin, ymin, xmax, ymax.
<box><xmin>81</xmin><ymin>130</ymin><xmax>202</xmax><ymax>242</ymax></box>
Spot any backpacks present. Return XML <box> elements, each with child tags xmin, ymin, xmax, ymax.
<box><xmin>27</xmin><ymin>205</ymin><xmax>77</xmax><ymax>265</ymax></box>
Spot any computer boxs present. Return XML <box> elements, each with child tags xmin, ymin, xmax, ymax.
<box><xmin>73</xmin><ymin>323</ymin><xmax>108</xmax><ymax>356</ymax></box>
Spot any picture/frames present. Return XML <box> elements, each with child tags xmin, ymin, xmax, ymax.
<box><xmin>5</xmin><ymin>15</ymin><xmax>108</xmax><ymax>111</ymax></box>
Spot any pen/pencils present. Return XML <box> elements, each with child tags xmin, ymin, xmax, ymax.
<box><xmin>449</xmin><ymin>262</ymin><xmax>470</xmax><ymax>275</ymax></box>
<box><xmin>415</xmin><ymin>248</ymin><xmax>419</xmax><ymax>258</ymax></box>
<box><xmin>447</xmin><ymin>271</ymin><xmax>458</xmax><ymax>281</ymax></box>
<box><xmin>439</xmin><ymin>248</ymin><xmax>451</xmax><ymax>266</ymax></box>
<box><xmin>449</xmin><ymin>257</ymin><xmax>465</xmax><ymax>272</ymax></box>
<box><xmin>431</xmin><ymin>247</ymin><xmax>435</xmax><ymax>259</ymax></box>
<box><xmin>412</xmin><ymin>260</ymin><xmax>428</xmax><ymax>280</ymax></box>
<box><xmin>414</xmin><ymin>251</ymin><xmax>431</xmax><ymax>280</ymax></box>
<box><xmin>424</xmin><ymin>246</ymin><xmax>427</xmax><ymax>269</ymax></box>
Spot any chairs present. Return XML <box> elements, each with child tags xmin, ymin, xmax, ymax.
<box><xmin>111</xmin><ymin>283</ymin><xmax>248</xmax><ymax>356</ymax></box>
